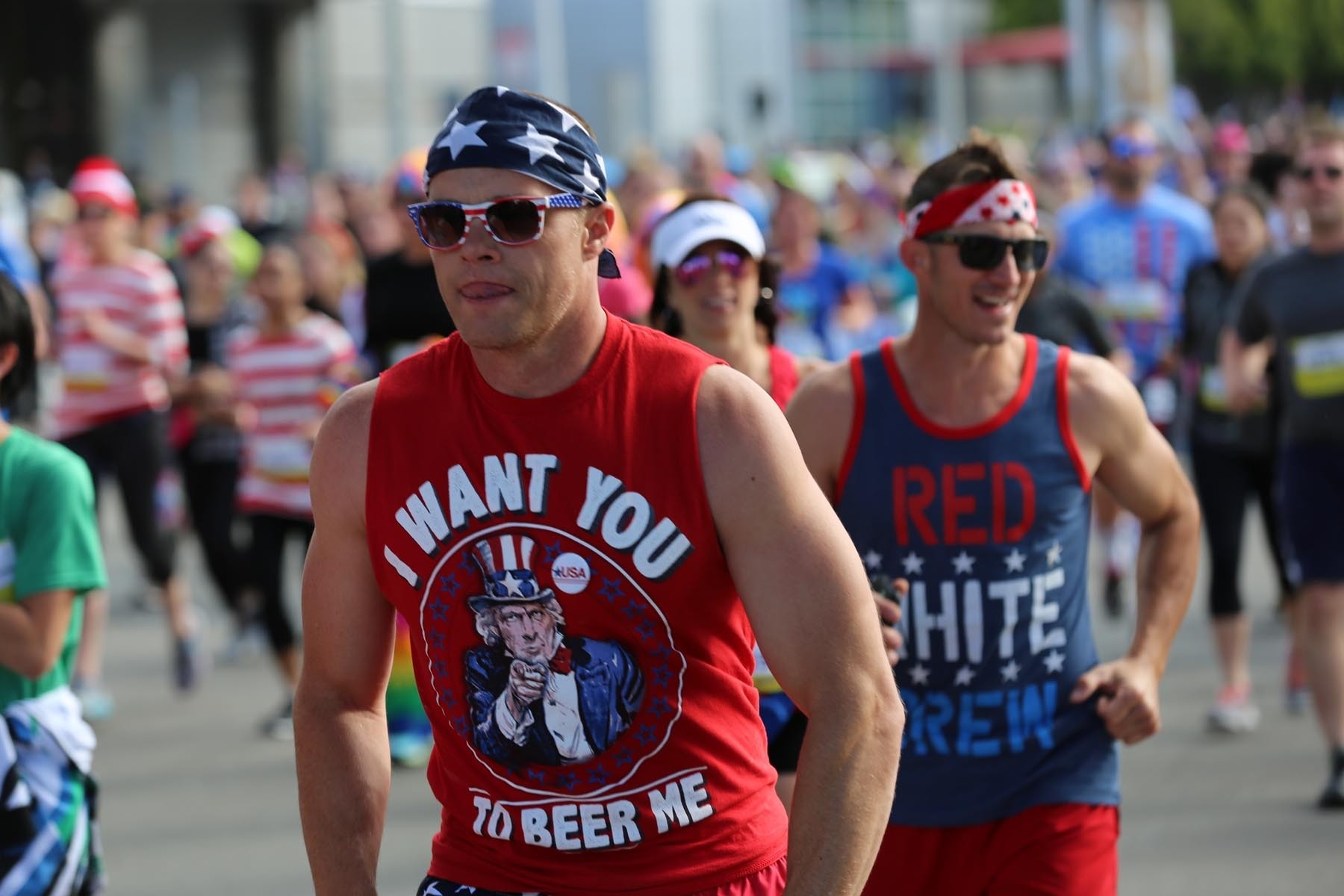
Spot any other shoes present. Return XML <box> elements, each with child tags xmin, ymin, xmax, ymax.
<box><xmin>174</xmin><ymin>638</ymin><xmax>194</xmax><ymax>689</ymax></box>
<box><xmin>1319</xmin><ymin>755</ymin><xmax>1344</xmax><ymax>808</ymax></box>
<box><xmin>390</xmin><ymin>717</ymin><xmax>433</xmax><ymax>765</ymax></box>
<box><xmin>1209</xmin><ymin>688</ymin><xmax>1260</xmax><ymax>732</ymax></box>
<box><xmin>1281</xmin><ymin>651</ymin><xmax>1314</xmax><ymax>713</ymax></box>
<box><xmin>1104</xmin><ymin>566</ymin><xmax>1126</xmax><ymax>619</ymax></box>
<box><xmin>74</xmin><ymin>677</ymin><xmax>107</xmax><ymax>722</ymax></box>
<box><xmin>263</xmin><ymin>703</ymin><xmax>293</xmax><ymax>740</ymax></box>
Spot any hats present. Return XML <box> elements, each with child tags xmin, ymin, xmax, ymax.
<box><xmin>649</xmin><ymin>200</ymin><xmax>766</xmax><ymax>273</ymax></box>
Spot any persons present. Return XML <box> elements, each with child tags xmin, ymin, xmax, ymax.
<box><xmin>290</xmin><ymin>87</ymin><xmax>906</xmax><ymax>896</ymax></box>
<box><xmin>0</xmin><ymin>87</ymin><xmax>1344</xmax><ymax>896</ymax></box>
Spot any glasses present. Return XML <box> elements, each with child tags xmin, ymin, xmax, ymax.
<box><xmin>918</xmin><ymin>232</ymin><xmax>1050</xmax><ymax>272</ymax></box>
<box><xmin>76</xmin><ymin>201</ymin><xmax>113</xmax><ymax>224</ymax></box>
<box><xmin>405</xmin><ymin>193</ymin><xmax>592</xmax><ymax>252</ymax></box>
<box><xmin>676</xmin><ymin>252</ymin><xmax>747</xmax><ymax>288</ymax></box>
<box><xmin>1296</xmin><ymin>167</ymin><xmax>1340</xmax><ymax>182</ymax></box>
